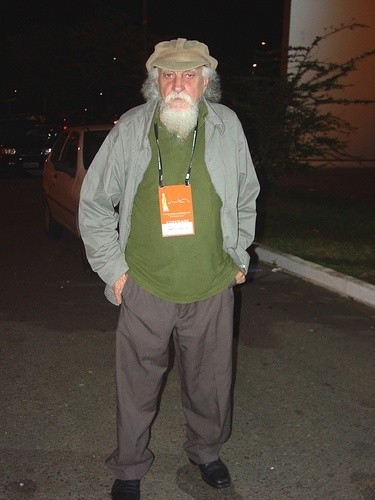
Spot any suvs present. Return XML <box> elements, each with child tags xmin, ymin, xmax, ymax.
<box><xmin>42</xmin><ymin>124</ymin><xmax>116</xmax><ymax>243</ymax></box>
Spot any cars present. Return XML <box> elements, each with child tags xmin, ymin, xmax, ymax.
<box><xmin>0</xmin><ymin>120</ymin><xmax>62</xmax><ymax>177</ymax></box>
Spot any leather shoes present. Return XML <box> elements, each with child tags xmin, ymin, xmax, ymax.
<box><xmin>110</xmin><ymin>479</ymin><xmax>141</xmax><ymax>500</ymax></box>
<box><xmin>188</xmin><ymin>455</ymin><xmax>232</xmax><ymax>489</ymax></box>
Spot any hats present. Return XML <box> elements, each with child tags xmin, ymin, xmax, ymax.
<box><xmin>145</xmin><ymin>37</ymin><xmax>218</xmax><ymax>75</ymax></box>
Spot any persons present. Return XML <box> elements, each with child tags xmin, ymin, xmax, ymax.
<box><xmin>77</xmin><ymin>38</ymin><xmax>261</xmax><ymax>500</ymax></box>
